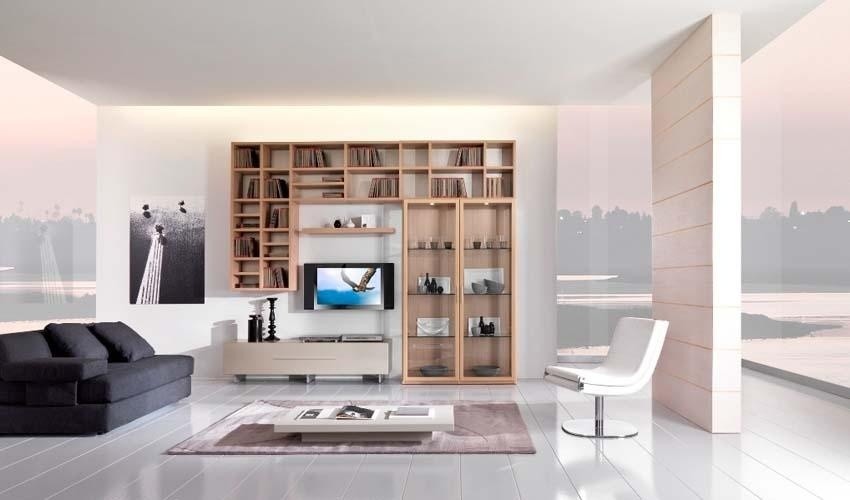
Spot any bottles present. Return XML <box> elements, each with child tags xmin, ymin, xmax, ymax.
<box><xmin>424</xmin><ymin>272</ymin><xmax>443</xmax><ymax>294</ymax></box>
<box><xmin>479</xmin><ymin>316</ymin><xmax>495</xmax><ymax>336</ymax></box>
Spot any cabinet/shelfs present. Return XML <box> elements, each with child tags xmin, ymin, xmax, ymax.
<box><xmin>224</xmin><ymin>338</ymin><xmax>392</xmax><ymax>383</ymax></box>
<box><xmin>228</xmin><ymin>141</ymin><xmax>519</xmax><ymax>386</ymax></box>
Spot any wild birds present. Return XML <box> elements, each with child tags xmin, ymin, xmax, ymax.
<box><xmin>340</xmin><ymin>267</ymin><xmax>376</xmax><ymax>292</ymax></box>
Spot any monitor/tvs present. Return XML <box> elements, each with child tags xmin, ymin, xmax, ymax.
<box><xmin>304</xmin><ymin>262</ymin><xmax>394</xmax><ymax>311</ymax></box>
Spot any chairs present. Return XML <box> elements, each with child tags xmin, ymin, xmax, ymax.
<box><xmin>544</xmin><ymin>315</ymin><xmax>669</xmax><ymax>439</ymax></box>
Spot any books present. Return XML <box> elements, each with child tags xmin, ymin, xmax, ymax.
<box><xmin>264</xmin><ymin>177</ymin><xmax>287</xmax><ymax>197</ymax></box>
<box><xmin>293</xmin><ymin>404</ymin><xmax>378</xmax><ymax>421</ymax></box>
<box><xmin>295</xmin><ymin>147</ymin><xmax>503</xmax><ymax>198</ymax></box>
<box><xmin>246</xmin><ymin>179</ymin><xmax>259</xmax><ymax>198</ymax></box>
<box><xmin>234</xmin><ymin>146</ymin><xmax>256</xmax><ymax>168</ymax></box>
<box><xmin>386</xmin><ymin>407</ymin><xmax>430</xmax><ymax>420</ymax></box>
<box><xmin>235</xmin><ymin>208</ymin><xmax>289</xmax><ymax>288</ymax></box>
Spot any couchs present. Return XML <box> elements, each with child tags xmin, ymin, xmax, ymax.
<box><xmin>0</xmin><ymin>321</ymin><xmax>194</xmax><ymax>435</ymax></box>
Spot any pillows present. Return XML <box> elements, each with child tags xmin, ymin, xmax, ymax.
<box><xmin>92</xmin><ymin>321</ymin><xmax>155</xmax><ymax>362</ymax></box>
<box><xmin>45</xmin><ymin>323</ymin><xmax>110</xmax><ymax>360</ymax></box>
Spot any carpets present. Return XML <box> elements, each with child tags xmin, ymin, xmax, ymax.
<box><xmin>165</xmin><ymin>397</ymin><xmax>536</xmax><ymax>460</ymax></box>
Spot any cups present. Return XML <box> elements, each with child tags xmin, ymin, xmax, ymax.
<box><xmin>418</xmin><ymin>241</ymin><xmax>451</xmax><ymax>248</ymax></box>
<box><xmin>473</xmin><ymin>240</ymin><xmax>507</xmax><ymax>248</ymax></box>
